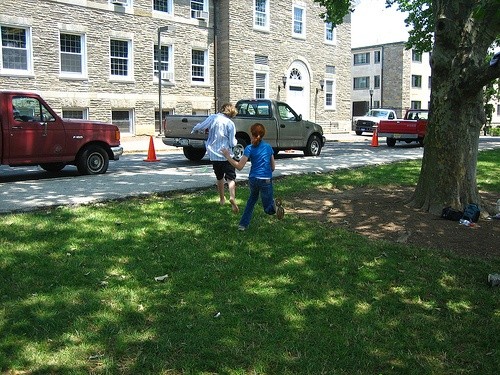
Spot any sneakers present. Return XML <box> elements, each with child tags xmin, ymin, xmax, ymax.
<box><xmin>238</xmin><ymin>225</ymin><xmax>250</xmax><ymax>231</ymax></box>
<box><xmin>274</xmin><ymin>197</ymin><xmax>284</xmax><ymax>220</ymax></box>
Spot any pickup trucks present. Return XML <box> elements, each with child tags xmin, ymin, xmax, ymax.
<box><xmin>378</xmin><ymin>108</ymin><xmax>429</xmax><ymax>145</ymax></box>
<box><xmin>161</xmin><ymin>97</ymin><xmax>326</xmax><ymax>162</ymax></box>
<box><xmin>354</xmin><ymin>109</ymin><xmax>404</xmax><ymax>136</ymax></box>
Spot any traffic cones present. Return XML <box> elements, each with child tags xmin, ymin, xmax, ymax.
<box><xmin>143</xmin><ymin>135</ymin><xmax>160</xmax><ymax>162</ymax></box>
<box><xmin>371</xmin><ymin>127</ymin><xmax>381</xmax><ymax>148</ymax></box>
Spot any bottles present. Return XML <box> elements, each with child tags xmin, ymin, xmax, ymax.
<box><xmin>459</xmin><ymin>219</ymin><xmax>472</xmax><ymax>226</ymax></box>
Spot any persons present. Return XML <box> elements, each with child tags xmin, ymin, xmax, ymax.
<box><xmin>191</xmin><ymin>104</ymin><xmax>239</xmax><ymax>213</ymax></box>
<box><xmin>221</xmin><ymin>122</ymin><xmax>285</xmax><ymax>231</ymax></box>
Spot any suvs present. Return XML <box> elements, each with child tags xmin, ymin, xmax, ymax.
<box><xmin>0</xmin><ymin>89</ymin><xmax>123</xmax><ymax>176</ymax></box>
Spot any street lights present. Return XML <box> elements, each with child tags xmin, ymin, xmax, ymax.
<box><xmin>156</xmin><ymin>25</ymin><xmax>169</xmax><ymax>134</ymax></box>
<box><xmin>368</xmin><ymin>88</ymin><xmax>374</xmax><ymax>110</ymax></box>
